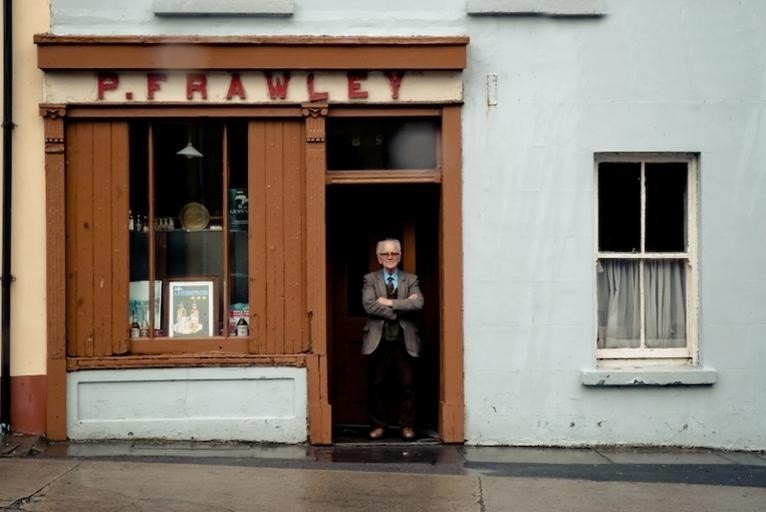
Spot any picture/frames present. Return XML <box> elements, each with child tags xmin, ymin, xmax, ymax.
<box><xmin>129</xmin><ymin>276</ymin><xmax>163</xmax><ymax>331</ymax></box>
<box><xmin>163</xmin><ymin>276</ymin><xmax>219</xmax><ymax>336</ymax></box>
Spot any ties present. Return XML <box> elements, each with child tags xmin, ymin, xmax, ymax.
<box><xmin>387</xmin><ymin>277</ymin><xmax>395</xmax><ymax>298</ymax></box>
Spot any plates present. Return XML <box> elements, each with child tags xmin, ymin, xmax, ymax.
<box><xmin>178</xmin><ymin>201</ymin><xmax>210</xmax><ymax>231</ymax></box>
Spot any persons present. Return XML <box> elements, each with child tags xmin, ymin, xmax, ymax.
<box><xmin>361</xmin><ymin>238</ymin><xmax>426</xmax><ymax>440</ymax></box>
<box><xmin>190</xmin><ymin>300</ymin><xmax>199</xmax><ymax>331</ymax></box>
<box><xmin>176</xmin><ymin>303</ymin><xmax>187</xmax><ymax>331</ymax></box>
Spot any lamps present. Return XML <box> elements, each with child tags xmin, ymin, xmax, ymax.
<box><xmin>176</xmin><ymin>118</ymin><xmax>204</xmax><ymax>159</ymax></box>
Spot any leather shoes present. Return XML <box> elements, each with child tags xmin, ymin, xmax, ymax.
<box><xmin>369</xmin><ymin>427</ymin><xmax>384</xmax><ymax>438</ymax></box>
<box><xmin>402</xmin><ymin>427</ymin><xmax>414</xmax><ymax>438</ymax></box>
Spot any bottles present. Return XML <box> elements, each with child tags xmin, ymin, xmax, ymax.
<box><xmin>132</xmin><ymin>309</ymin><xmax>141</xmax><ymax>338</ymax></box>
<box><xmin>229</xmin><ymin>310</ymin><xmax>248</xmax><ymax>338</ymax></box>
<box><xmin>130</xmin><ymin>209</ymin><xmax>147</xmax><ymax>231</ymax></box>
